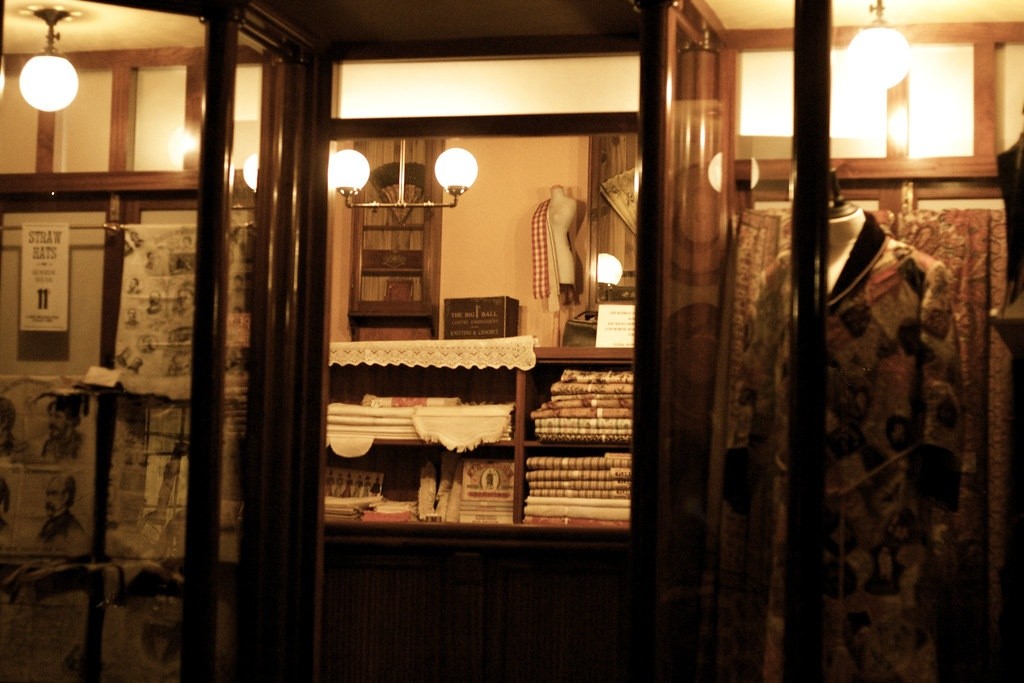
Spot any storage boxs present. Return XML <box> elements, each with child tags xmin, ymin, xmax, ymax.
<box><xmin>443</xmin><ymin>294</ymin><xmax>519</xmax><ymax>341</ymax></box>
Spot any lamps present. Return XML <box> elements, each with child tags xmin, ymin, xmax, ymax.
<box><xmin>19</xmin><ymin>10</ymin><xmax>80</xmax><ymax>113</ymax></box>
<box><xmin>330</xmin><ymin>137</ymin><xmax>479</xmax><ymax>208</ymax></box>
<box><xmin>597</xmin><ymin>253</ymin><xmax>622</xmax><ymax>300</ymax></box>
<box><xmin>848</xmin><ymin>0</ymin><xmax>911</xmax><ymax>90</ymax></box>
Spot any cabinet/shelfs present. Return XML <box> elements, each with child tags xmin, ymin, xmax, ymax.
<box><xmin>324</xmin><ymin>334</ymin><xmax>635</xmax><ymax>530</ymax></box>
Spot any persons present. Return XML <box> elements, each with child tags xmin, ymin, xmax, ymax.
<box><xmin>546</xmin><ymin>185</ymin><xmax>577</xmax><ymax>285</ymax></box>
<box><xmin>753</xmin><ymin>205</ymin><xmax>962</xmax><ymax>683</ymax></box>
<box><xmin>326</xmin><ymin>471</ymin><xmax>381</xmax><ymax>497</ymax></box>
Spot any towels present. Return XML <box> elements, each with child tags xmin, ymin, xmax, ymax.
<box><xmin>327</xmin><ymin>393</ymin><xmax>513</xmax><ymax>454</ymax></box>
<box><xmin>530</xmin><ymin>369</ymin><xmax>634</xmax><ymax>444</ymax></box>
<box><xmin>524</xmin><ymin>452</ymin><xmax>630</xmax><ymax>529</ymax></box>
<box><xmin>324</xmin><ymin>451</ymin><xmax>514</xmax><ymax>523</ymax></box>
